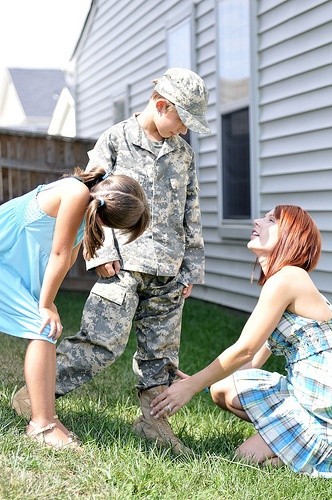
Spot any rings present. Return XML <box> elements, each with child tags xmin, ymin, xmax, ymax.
<box><xmin>167</xmin><ymin>405</ymin><xmax>171</xmax><ymax>412</ymax></box>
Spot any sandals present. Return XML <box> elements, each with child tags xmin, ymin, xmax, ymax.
<box><xmin>25</xmin><ymin>414</ymin><xmax>85</xmax><ymax>452</ymax></box>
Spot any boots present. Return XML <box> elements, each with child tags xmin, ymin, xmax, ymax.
<box><xmin>11</xmin><ymin>385</ymin><xmax>31</xmax><ymax>421</ymax></box>
<box><xmin>128</xmin><ymin>386</ymin><xmax>192</xmax><ymax>457</ymax></box>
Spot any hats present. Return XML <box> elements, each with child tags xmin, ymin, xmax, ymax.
<box><xmin>153</xmin><ymin>68</ymin><xmax>213</xmax><ymax>135</ymax></box>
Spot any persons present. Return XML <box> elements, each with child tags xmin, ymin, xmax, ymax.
<box><xmin>7</xmin><ymin>68</ymin><xmax>212</xmax><ymax>459</ymax></box>
<box><xmin>150</xmin><ymin>205</ymin><xmax>332</xmax><ymax>479</ymax></box>
<box><xmin>0</xmin><ymin>167</ymin><xmax>150</xmax><ymax>453</ymax></box>
